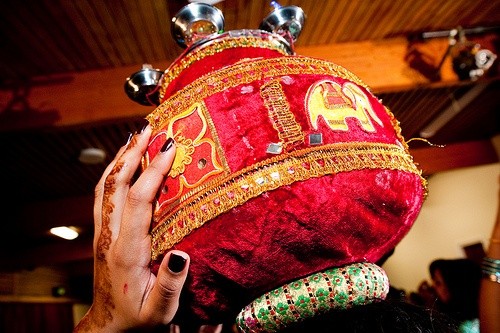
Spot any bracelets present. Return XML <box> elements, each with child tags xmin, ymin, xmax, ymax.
<box><xmin>482</xmin><ymin>255</ymin><xmax>500</xmax><ymax>284</ymax></box>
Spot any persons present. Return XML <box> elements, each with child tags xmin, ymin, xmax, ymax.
<box><xmin>67</xmin><ymin>118</ymin><xmax>500</xmax><ymax>333</ymax></box>
